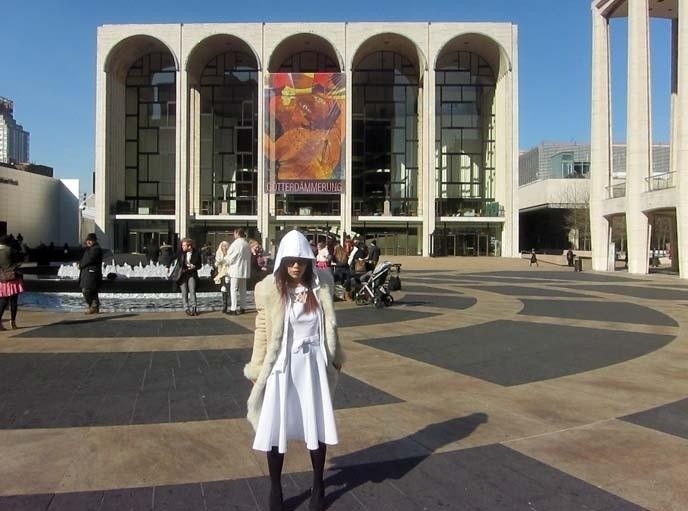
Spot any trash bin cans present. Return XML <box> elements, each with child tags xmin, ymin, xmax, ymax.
<box><xmin>575</xmin><ymin>260</ymin><xmax>582</xmax><ymax>272</ymax></box>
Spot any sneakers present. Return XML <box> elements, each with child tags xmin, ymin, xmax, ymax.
<box><xmin>226</xmin><ymin>308</ymin><xmax>245</xmax><ymax>315</ymax></box>
<box><xmin>187</xmin><ymin>306</ymin><xmax>198</xmax><ymax>316</ymax></box>
<box><xmin>86</xmin><ymin>300</ymin><xmax>99</xmax><ymax>314</ymax></box>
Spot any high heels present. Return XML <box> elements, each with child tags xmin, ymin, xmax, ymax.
<box><xmin>10</xmin><ymin>319</ymin><xmax>17</xmax><ymax>329</ymax></box>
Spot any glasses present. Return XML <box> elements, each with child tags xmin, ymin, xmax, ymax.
<box><xmin>284</xmin><ymin>258</ymin><xmax>308</xmax><ymax>267</ymax></box>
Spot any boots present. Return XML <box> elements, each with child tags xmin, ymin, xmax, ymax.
<box><xmin>268</xmin><ymin>446</ymin><xmax>285</xmax><ymax>511</ymax></box>
<box><xmin>222</xmin><ymin>292</ymin><xmax>227</xmax><ymax>313</ymax></box>
<box><xmin>309</xmin><ymin>442</ymin><xmax>327</xmax><ymax>510</ymax></box>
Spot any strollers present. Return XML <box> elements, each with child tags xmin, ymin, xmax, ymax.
<box><xmin>356</xmin><ymin>261</ymin><xmax>401</xmax><ymax>305</ymax></box>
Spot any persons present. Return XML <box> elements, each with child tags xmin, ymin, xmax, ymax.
<box><xmin>566</xmin><ymin>248</ymin><xmax>576</xmax><ymax>266</ymax></box>
<box><xmin>309</xmin><ymin>236</ymin><xmax>381</xmax><ymax>266</ymax></box>
<box><xmin>530</xmin><ymin>248</ymin><xmax>539</xmax><ymax>267</ymax></box>
<box><xmin>79</xmin><ymin>233</ymin><xmax>102</xmax><ymax>315</ymax></box>
<box><xmin>242</xmin><ymin>229</ymin><xmax>347</xmax><ymax>511</ymax></box>
<box><xmin>271</xmin><ymin>239</ymin><xmax>276</xmax><ymax>259</ymax></box>
<box><xmin>0</xmin><ymin>233</ymin><xmax>25</xmax><ymax>331</ymax></box>
<box><xmin>147</xmin><ymin>228</ymin><xmax>259</xmax><ymax>316</ymax></box>
